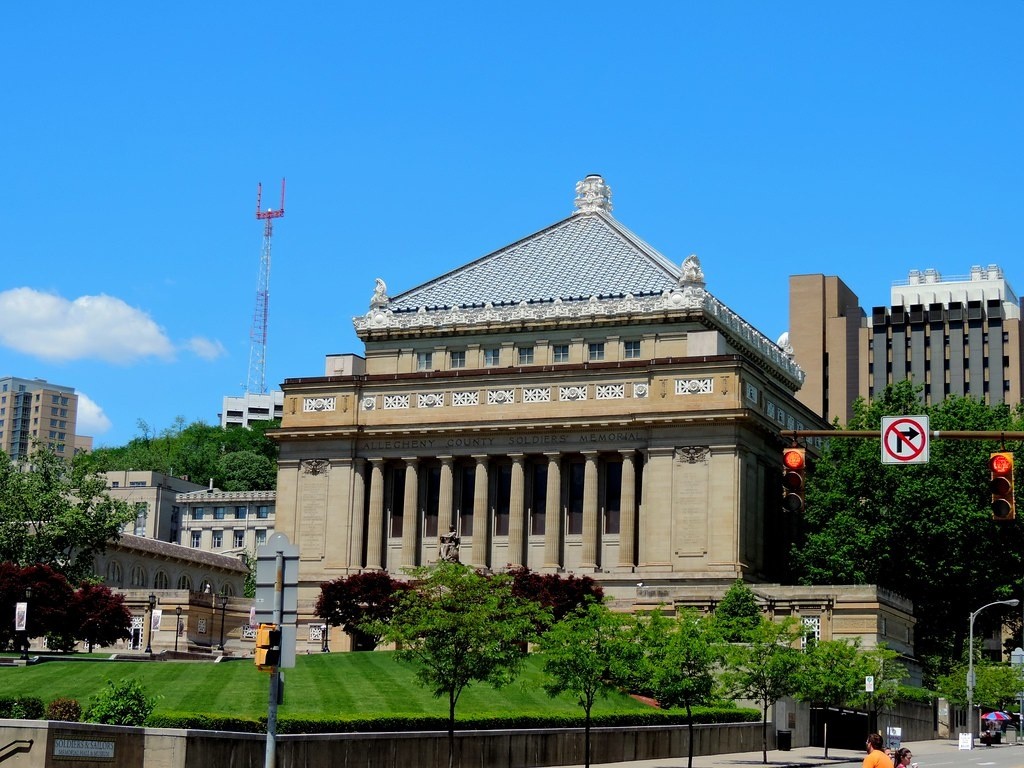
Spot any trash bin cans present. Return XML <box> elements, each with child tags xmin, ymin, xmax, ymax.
<box><xmin>776</xmin><ymin>728</ymin><xmax>792</xmax><ymax>752</ymax></box>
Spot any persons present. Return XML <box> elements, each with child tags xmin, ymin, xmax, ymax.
<box><xmin>861</xmin><ymin>734</ymin><xmax>895</xmax><ymax>768</ymax></box>
<box><xmin>882</xmin><ymin>746</ymin><xmax>893</xmax><ymax>761</ymax></box>
<box><xmin>894</xmin><ymin>747</ymin><xmax>918</xmax><ymax>768</ymax></box>
<box><xmin>439</xmin><ymin>525</ymin><xmax>463</xmax><ymax>566</ymax></box>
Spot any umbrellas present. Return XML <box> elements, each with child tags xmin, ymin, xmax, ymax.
<box><xmin>980</xmin><ymin>711</ymin><xmax>1012</xmax><ymax>732</ymax></box>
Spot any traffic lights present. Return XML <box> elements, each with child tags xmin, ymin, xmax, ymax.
<box><xmin>254</xmin><ymin>623</ymin><xmax>281</xmax><ymax>673</ymax></box>
<box><xmin>781</xmin><ymin>448</ymin><xmax>806</xmax><ymax>515</ymax></box>
<box><xmin>989</xmin><ymin>452</ymin><xmax>1016</xmax><ymax>522</ymax></box>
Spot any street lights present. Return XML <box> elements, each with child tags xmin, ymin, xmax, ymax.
<box><xmin>144</xmin><ymin>592</ymin><xmax>157</xmax><ymax>654</ymax></box>
<box><xmin>173</xmin><ymin>605</ymin><xmax>183</xmax><ymax>652</ymax></box>
<box><xmin>969</xmin><ymin>599</ymin><xmax>1019</xmax><ymax>750</ymax></box>
<box><xmin>218</xmin><ymin>593</ymin><xmax>229</xmax><ymax>651</ymax></box>
